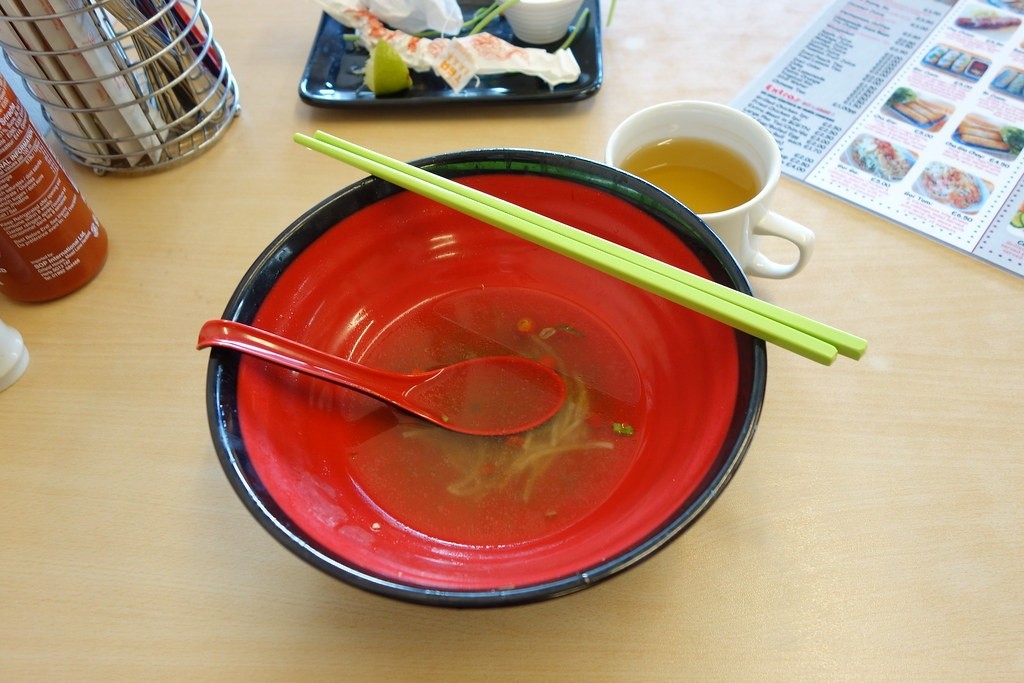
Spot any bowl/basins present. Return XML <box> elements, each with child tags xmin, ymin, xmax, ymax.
<box><xmin>205</xmin><ymin>147</ymin><xmax>768</xmax><ymax>611</ymax></box>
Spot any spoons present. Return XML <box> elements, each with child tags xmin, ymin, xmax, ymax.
<box><xmin>196</xmin><ymin>319</ymin><xmax>567</xmax><ymax>438</ymax></box>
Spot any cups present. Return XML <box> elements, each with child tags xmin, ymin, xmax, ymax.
<box><xmin>606</xmin><ymin>98</ymin><xmax>816</xmax><ymax>280</ymax></box>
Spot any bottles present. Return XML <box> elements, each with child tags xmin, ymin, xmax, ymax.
<box><xmin>0</xmin><ymin>74</ymin><xmax>108</xmax><ymax>304</ymax></box>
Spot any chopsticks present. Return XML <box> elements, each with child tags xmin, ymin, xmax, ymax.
<box><xmin>291</xmin><ymin>129</ymin><xmax>870</xmax><ymax>366</ymax></box>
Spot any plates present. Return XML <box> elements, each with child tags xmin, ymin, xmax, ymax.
<box><xmin>844</xmin><ymin>2</ymin><xmax>1023</xmax><ymax>212</ymax></box>
<box><xmin>299</xmin><ymin>0</ymin><xmax>603</xmax><ymax>114</ymax></box>
